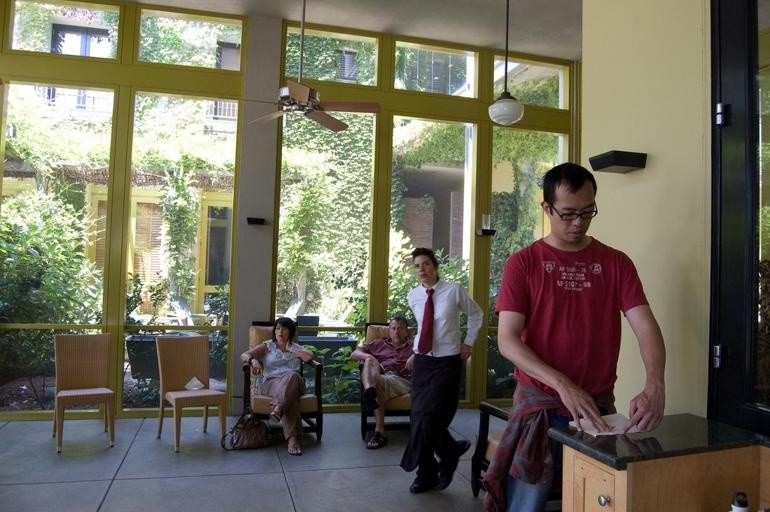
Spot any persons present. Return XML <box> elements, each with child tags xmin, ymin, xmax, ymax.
<box><xmin>400</xmin><ymin>247</ymin><xmax>484</xmax><ymax>494</ymax></box>
<box><xmin>495</xmin><ymin>163</ymin><xmax>667</xmax><ymax>512</ymax></box>
<box><xmin>240</xmin><ymin>317</ymin><xmax>314</xmax><ymax>455</ymax></box>
<box><xmin>350</xmin><ymin>314</ymin><xmax>419</xmax><ymax>449</ymax></box>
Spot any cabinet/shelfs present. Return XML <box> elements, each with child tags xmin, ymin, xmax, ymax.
<box><xmin>545</xmin><ymin>410</ymin><xmax>758</xmax><ymax>511</ymax></box>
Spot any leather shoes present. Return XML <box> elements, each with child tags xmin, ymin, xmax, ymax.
<box><xmin>410</xmin><ymin>476</ymin><xmax>439</xmax><ymax>493</ymax></box>
<box><xmin>438</xmin><ymin>459</ymin><xmax>459</xmax><ymax>489</ymax></box>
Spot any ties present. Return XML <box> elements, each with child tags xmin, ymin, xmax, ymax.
<box><xmin>418</xmin><ymin>290</ymin><xmax>434</xmax><ymax>355</ymax></box>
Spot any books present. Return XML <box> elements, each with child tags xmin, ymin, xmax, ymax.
<box><xmin>569</xmin><ymin>413</ymin><xmax>640</xmax><ymax>438</ymax></box>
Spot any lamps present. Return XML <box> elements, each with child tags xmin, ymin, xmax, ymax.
<box><xmin>487</xmin><ymin>0</ymin><xmax>526</xmax><ymax>129</ymax></box>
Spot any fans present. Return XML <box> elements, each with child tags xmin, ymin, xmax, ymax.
<box><xmin>240</xmin><ymin>0</ymin><xmax>383</xmax><ymax>134</ymax></box>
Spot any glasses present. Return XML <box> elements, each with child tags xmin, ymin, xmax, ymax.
<box><xmin>550</xmin><ymin>200</ymin><xmax>598</xmax><ymax>221</ymax></box>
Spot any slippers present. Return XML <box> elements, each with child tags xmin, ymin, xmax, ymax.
<box><xmin>367</xmin><ymin>432</ymin><xmax>388</xmax><ymax>449</ymax></box>
<box><xmin>269</xmin><ymin>410</ymin><xmax>281</xmax><ymax>424</ymax></box>
<box><xmin>363</xmin><ymin>387</ymin><xmax>380</xmax><ymax>411</ymax></box>
<box><xmin>286</xmin><ymin>441</ymin><xmax>302</xmax><ymax>455</ymax></box>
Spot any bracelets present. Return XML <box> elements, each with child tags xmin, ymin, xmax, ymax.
<box><xmin>248</xmin><ymin>357</ymin><xmax>254</xmax><ymax>365</ymax></box>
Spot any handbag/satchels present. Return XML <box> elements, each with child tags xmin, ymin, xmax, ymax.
<box><xmin>221</xmin><ymin>406</ymin><xmax>275</xmax><ymax>451</ymax></box>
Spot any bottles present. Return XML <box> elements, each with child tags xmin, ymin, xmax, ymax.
<box><xmin>256</xmin><ymin>375</ymin><xmax>264</xmax><ymax>396</ymax></box>
<box><xmin>730</xmin><ymin>490</ymin><xmax>749</xmax><ymax>512</ymax></box>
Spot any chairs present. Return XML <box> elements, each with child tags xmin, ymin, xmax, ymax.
<box><xmin>165</xmin><ymin>308</ymin><xmax>322</xmax><ymax>337</ymax></box>
<box><xmin>48</xmin><ymin>331</ymin><xmax>117</xmax><ymax>455</ymax></box>
<box><xmin>153</xmin><ymin>334</ymin><xmax>229</xmax><ymax>454</ymax></box>
<box><xmin>358</xmin><ymin>321</ymin><xmax>420</xmax><ymax>442</ymax></box>
<box><xmin>469</xmin><ymin>399</ymin><xmax>512</xmax><ymax>499</ymax></box>
<box><xmin>239</xmin><ymin>324</ymin><xmax>326</xmax><ymax>443</ymax></box>
